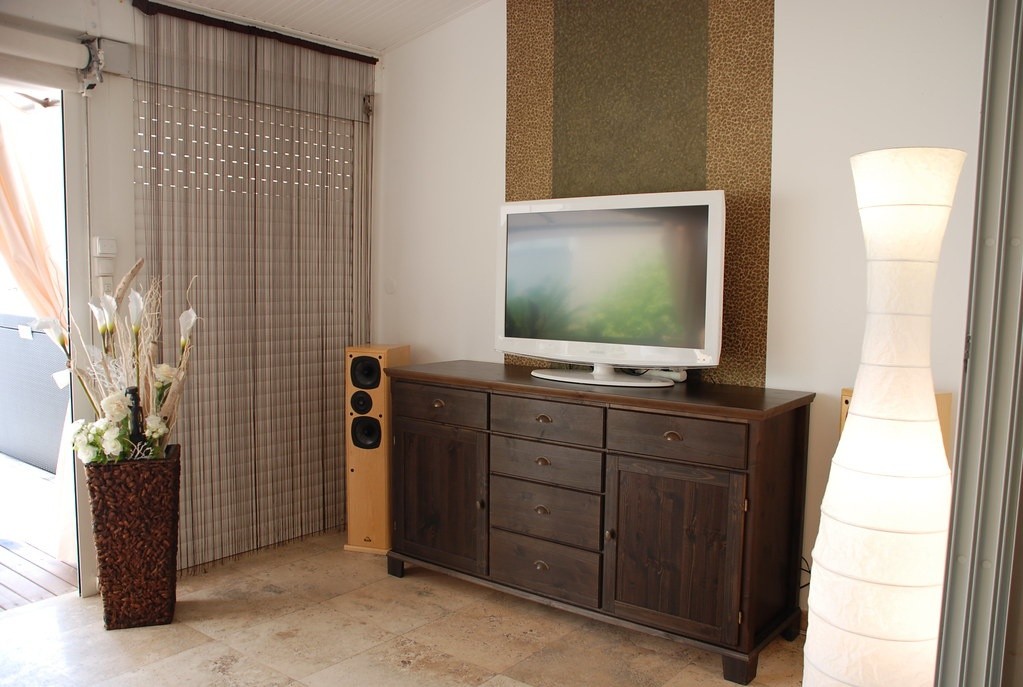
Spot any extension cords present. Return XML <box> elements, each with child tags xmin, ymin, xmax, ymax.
<box><xmin>635</xmin><ymin>369</ymin><xmax>687</xmax><ymax>383</ymax></box>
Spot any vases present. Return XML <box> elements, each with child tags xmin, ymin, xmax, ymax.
<box><xmin>84</xmin><ymin>444</ymin><xmax>181</xmax><ymax>630</ymax></box>
<box><xmin>803</xmin><ymin>147</ymin><xmax>970</xmax><ymax>687</ymax></box>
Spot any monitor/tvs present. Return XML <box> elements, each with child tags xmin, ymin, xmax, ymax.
<box><xmin>493</xmin><ymin>189</ymin><xmax>727</xmax><ymax>387</ymax></box>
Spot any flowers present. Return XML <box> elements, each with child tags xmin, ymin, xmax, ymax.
<box><xmin>37</xmin><ymin>273</ymin><xmax>206</xmax><ymax>464</ymax></box>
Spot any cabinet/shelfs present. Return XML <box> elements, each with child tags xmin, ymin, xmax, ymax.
<box><xmin>383</xmin><ymin>360</ymin><xmax>816</xmax><ymax>687</ymax></box>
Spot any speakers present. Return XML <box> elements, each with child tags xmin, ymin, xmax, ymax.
<box><xmin>339</xmin><ymin>345</ymin><xmax>413</xmax><ymax>555</ymax></box>
<box><xmin>839</xmin><ymin>387</ymin><xmax>953</xmax><ymax>466</ymax></box>
